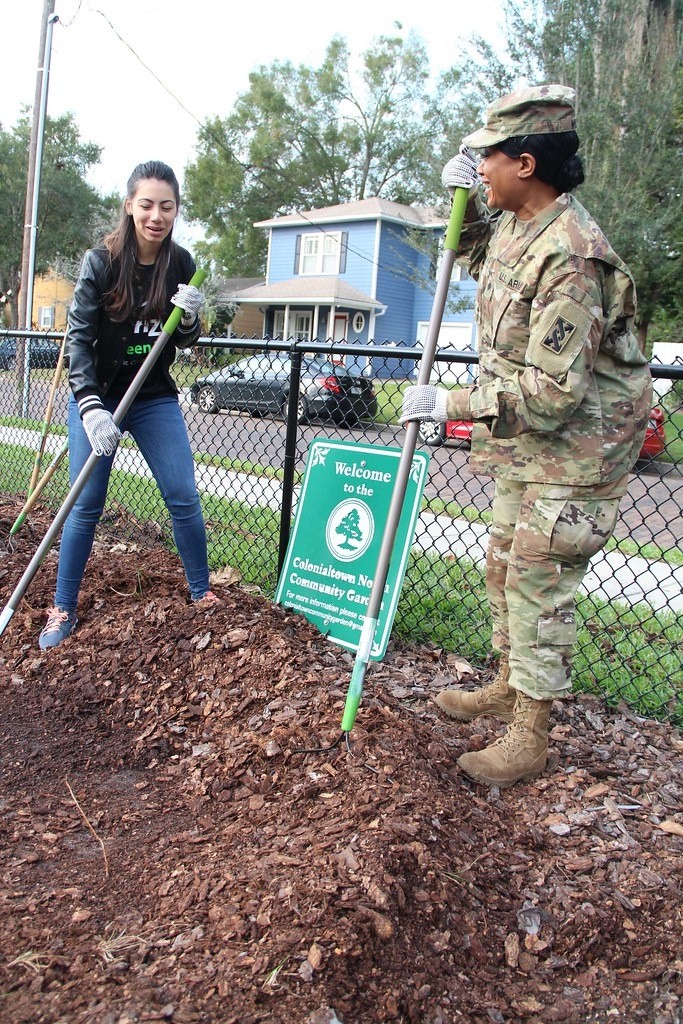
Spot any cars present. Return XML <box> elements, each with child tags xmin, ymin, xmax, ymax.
<box><xmin>190</xmin><ymin>353</ymin><xmax>377</xmax><ymax>427</ymax></box>
<box><xmin>0</xmin><ymin>336</ymin><xmax>67</xmax><ymax>370</ymax></box>
<box><xmin>404</xmin><ymin>381</ymin><xmax>664</xmax><ymax>469</ymax></box>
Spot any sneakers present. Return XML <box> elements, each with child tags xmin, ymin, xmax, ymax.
<box><xmin>39</xmin><ymin>606</ymin><xmax>78</xmax><ymax>649</ymax></box>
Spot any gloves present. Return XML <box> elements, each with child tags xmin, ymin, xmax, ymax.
<box><xmin>397</xmin><ymin>385</ymin><xmax>450</xmax><ymax>425</ymax></box>
<box><xmin>81</xmin><ymin>408</ymin><xmax>122</xmax><ymax>456</ymax></box>
<box><xmin>442</xmin><ymin>144</ymin><xmax>479</xmax><ymax>200</ymax></box>
<box><xmin>170</xmin><ymin>283</ymin><xmax>203</xmax><ymax>326</ymax></box>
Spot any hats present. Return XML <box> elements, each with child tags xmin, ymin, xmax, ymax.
<box><xmin>462</xmin><ymin>84</ymin><xmax>576</xmax><ymax>148</ymax></box>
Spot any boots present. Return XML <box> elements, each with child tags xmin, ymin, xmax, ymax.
<box><xmin>434</xmin><ymin>656</ymin><xmax>517</xmax><ymax>723</ymax></box>
<box><xmin>457</xmin><ymin>689</ymin><xmax>554</xmax><ymax>788</ymax></box>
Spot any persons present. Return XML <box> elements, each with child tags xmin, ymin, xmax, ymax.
<box><xmin>400</xmin><ymin>86</ymin><xmax>652</xmax><ymax>788</ymax></box>
<box><xmin>39</xmin><ymin>160</ymin><xmax>220</xmax><ymax>650</ymax></box>
